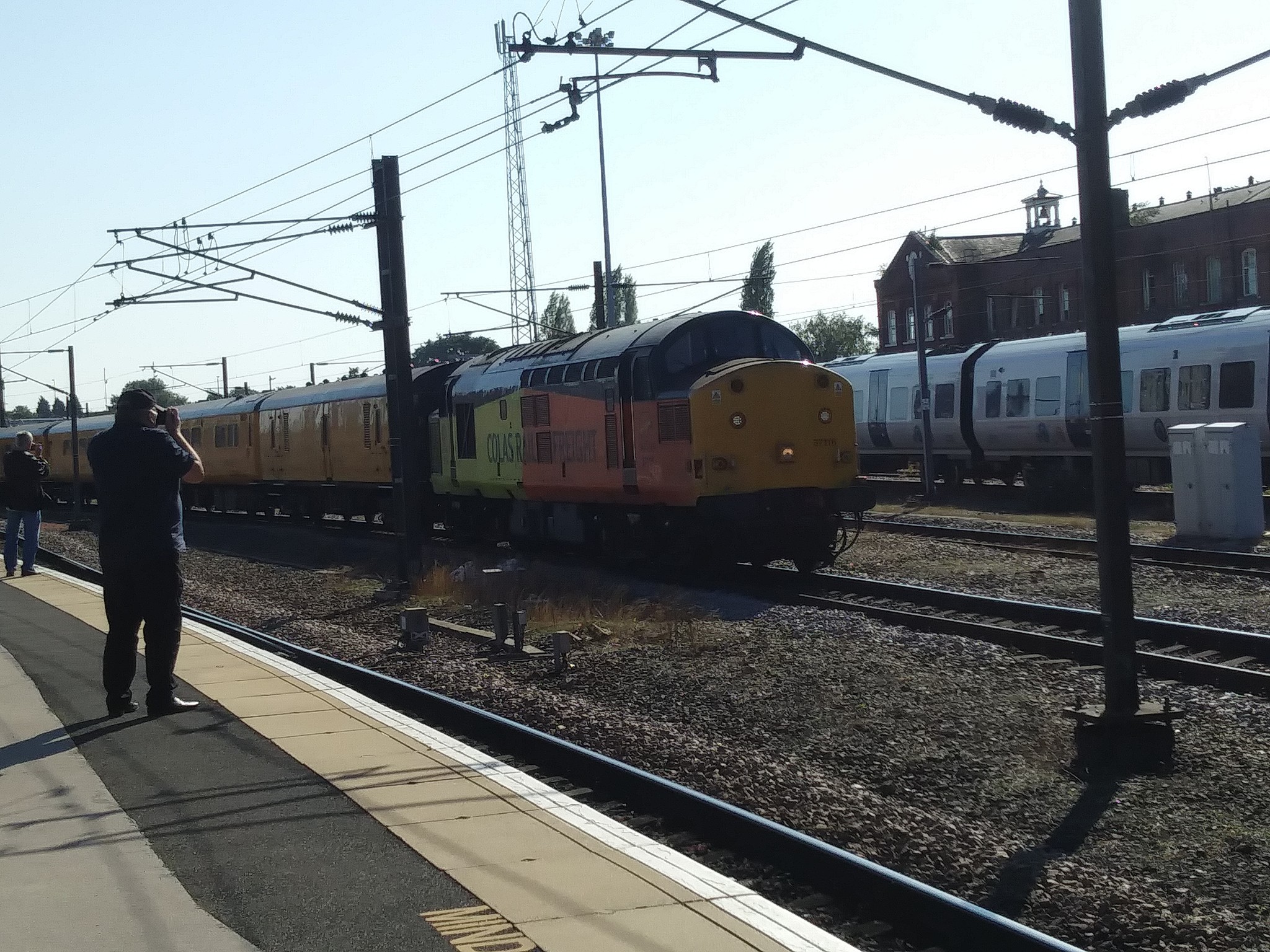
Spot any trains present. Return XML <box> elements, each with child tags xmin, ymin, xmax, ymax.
<box><xmin>818</xmin><ymin>304</ymin><xmax>1270</xmax><ymax>487</ymax></box>
<box><xmin>0</xmin><ymin>310</ymin><xmax>858</xmax><ymax>576</ymax></box>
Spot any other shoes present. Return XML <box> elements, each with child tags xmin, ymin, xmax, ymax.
<box><xmin>146</xmin><ymin>696</ymin><xmax>200</xmax><ymax>717</ymax></box>
<box><xmin>21</xmin><ymin>568</ymin><xmax>41</xmax><ymax>576</ymax></box>
<box><xmin>6</xmin><ymin>570</ymin><xmax>17</xmax><ymax>576</ymax></box>
<box><xmin>104</xmin><ymin>699</ymin><xmax>140</xmax><ymax>719</ymax></box>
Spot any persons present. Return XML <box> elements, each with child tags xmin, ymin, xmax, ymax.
<box><xmin>86</xmin><ymin>389</ymin><xmax>205</xmax><ymax>716</ymax></box>
<box><xmin>4</xmin><ymin>431</ymin><xmax>50</xmax><ymax>578</ymax></box>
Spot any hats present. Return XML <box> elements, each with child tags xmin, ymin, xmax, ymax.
<box><xmin>116</xmin><ymin>388</ymin><xmax>167</xmax><ymax>412</ymax></box>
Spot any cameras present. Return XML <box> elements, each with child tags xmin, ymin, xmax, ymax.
<box><xmin>31</xmin><ymin>445</ymin><xmax>36</xmax><ymax>451</ymax></box>
<box><xmin>156</xmin><ymin>411</ymin><xmax>168</xmax><ymax>425</ymax></box>
